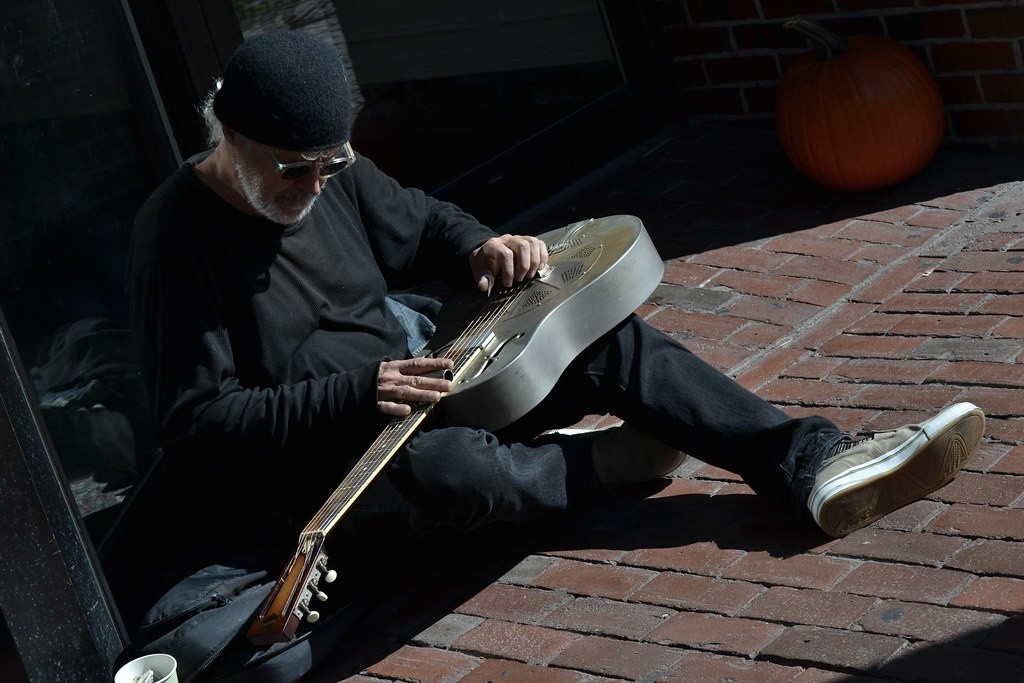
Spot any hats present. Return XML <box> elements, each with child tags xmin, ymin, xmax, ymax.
<box><xmin>213</xmin><ymin>30</ymin><xmax>351</xmax><ymax>150</ymax></box>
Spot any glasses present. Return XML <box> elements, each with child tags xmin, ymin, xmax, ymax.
<box><xmin>263</xmin><ymin>142</ymin><xmax>356</xmax><ymax>180</ymax></box>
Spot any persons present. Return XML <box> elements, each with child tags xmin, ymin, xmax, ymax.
<box><xmin>128</xmin><ymin>32</ymin><xmax>986</xmax><ymax>548</ymax></box>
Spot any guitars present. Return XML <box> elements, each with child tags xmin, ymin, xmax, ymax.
<box><xmin>245</xmin><ymin>214</ymin><xmax>666</xmax><ymax>648</ymax></box>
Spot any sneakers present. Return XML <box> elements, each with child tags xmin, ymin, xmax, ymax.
<box><xmin>808</xmin><ymin>401</ymin><xmax>986</xmax><ymax>537</ymax></box>
<box><xmin>591</xmin><ymin>422</ymin><xmax>687</xmax><ymax>487</ymax></box>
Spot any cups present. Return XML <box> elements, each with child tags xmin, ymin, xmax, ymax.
<box><xmin>114</xmin><ymin>654</ymin><xmax>178</xmax><ymax>683</ymax></box>
